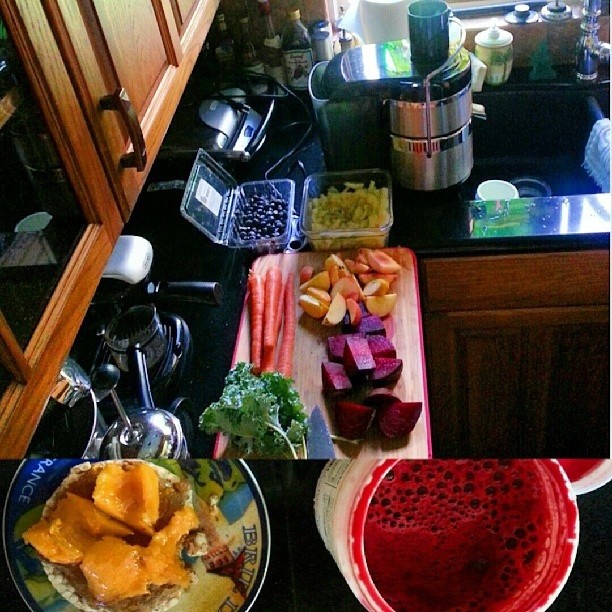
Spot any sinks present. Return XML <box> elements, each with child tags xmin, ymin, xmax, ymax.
<box><xmin>454</xmin><ymin>83</ymin><xmax>610</xmax><ymax>203</ymax></box>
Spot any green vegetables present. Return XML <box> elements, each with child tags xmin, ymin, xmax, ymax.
<box><xmin>199</xmin><ymin>362</ymin><xmax>306</xmax><ymax>460</ymax></box>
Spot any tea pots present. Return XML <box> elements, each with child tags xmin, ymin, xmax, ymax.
<box><xmin>91</xmin><ymin>342</ymin><xmax>189</xmax><ymax>459</ymax></box>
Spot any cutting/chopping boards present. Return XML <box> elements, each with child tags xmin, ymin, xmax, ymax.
<box><xmin>214</xmin><ymin>248</ymin><xmax>435</xmax><ymax>461</ymax></box>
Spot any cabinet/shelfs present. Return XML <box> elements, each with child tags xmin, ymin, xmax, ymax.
<box><xmin>0</xmin><ymin>0</ymin><xmax>123</xmax><ymax>460</ymax></box>
<box><xmin>426</xmin><ymin>251</ymin><xmax>609</xmax><ymax>456</ymax></box>
<box><xmin>39</xmin><ymin>0</ymin><xmax>183</xmax><ymax>223</ymax></box>
<box><xmin>171</xmin><ymin>0</ymin><xmax>220</xmax><ymax>71</ymax></box>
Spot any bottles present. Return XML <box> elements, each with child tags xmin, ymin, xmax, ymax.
<box><xmin>313</xmin><ymin>458</ymin><xmax>580</xmax><ymax>612</ymax></box>
<box><xmin>214</xmin><ymin>13</ymin><xmax>236</xmax><ymax>88</ymax></box>
<box><xmin>281</xmin><ymin>8</ymin><xmax>314</xmax><ymax>91</ymax></box>
<box><xmin>194</xmin><ymin>43</ymin><xmax>215</xmax><ymax>98</ymax></box>
<box><xmin>237</xmin><ymin>15</ymin><xmax>268</xmax><ymax>95</ymax></box>
<box><xmin>256</xmin><ymin>0</ymin><xmax>288</xmax><ymax>93</ymax></box>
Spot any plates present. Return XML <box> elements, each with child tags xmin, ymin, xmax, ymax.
<box><xmin>3</xmin><ymin>460</ymin><xmax>273</xmax><ymax>612</ymax></box>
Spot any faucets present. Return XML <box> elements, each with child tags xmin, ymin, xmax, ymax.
<box><xmin>576</xmin><ymin>1</ymin><xmax>610</xmax><ymax>81</ymax></box>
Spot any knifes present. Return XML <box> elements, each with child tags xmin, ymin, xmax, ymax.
<box><xmin>306</xmin><ymin>404</ymin><xmax>336</xmax><ymax>459</ymax></box>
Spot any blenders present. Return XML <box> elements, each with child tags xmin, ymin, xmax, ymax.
<box><xmin>308</xmin><ymin>1</ymin><xmax>474</xmax><ymax>194</ymax></box>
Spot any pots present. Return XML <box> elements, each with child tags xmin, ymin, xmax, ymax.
<box><xmin>26</xmin><ymin>377</ymin><xmax>109</xmax><ymax>459</ymax></box>
<box><xmin>96</xmin><ymin>303</ymin><xmax>168</xmax><ymax>374</ymax></box>
<box><xmin>90</xmin><ymin>235</ymin><xmax>223</xmax><ymax>308</ymax></box>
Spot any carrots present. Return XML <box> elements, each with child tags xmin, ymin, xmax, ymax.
<box><xmin>246</xmin><ymin>266</ymin><xmax>295</xmax><ymax>376</ymax></box>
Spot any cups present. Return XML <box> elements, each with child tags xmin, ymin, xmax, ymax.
<box><xmin>476</xmin><ymin>179</ymin><xmax>520</xmax><ymax>200</ymax></box>
